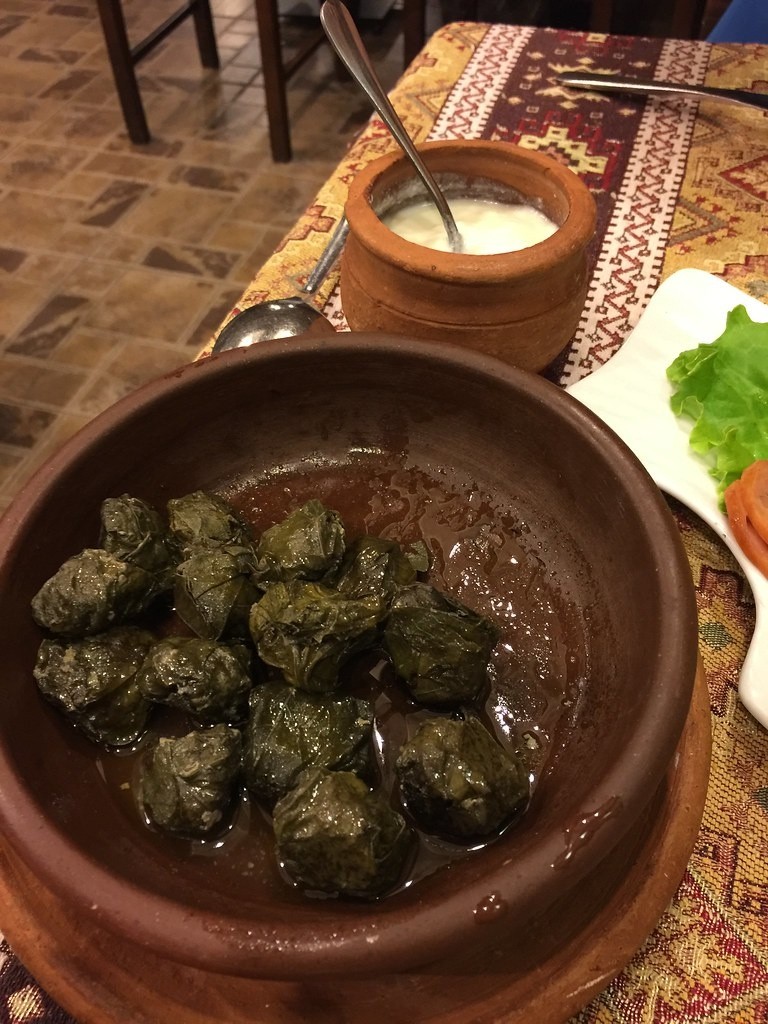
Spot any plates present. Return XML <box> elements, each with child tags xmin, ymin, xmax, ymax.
<box><xmin>565</xmin><ymin>268</ymin><xmax>768</xmax><ymax>736</ymax></box>
<box><xmin>0</xmin><ymin>659</ymin><xmax>710</xmax><ymax>1023</ymax></box>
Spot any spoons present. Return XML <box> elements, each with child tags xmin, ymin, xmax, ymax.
<box><xmin>212</xmin><ymin>218</ymin><xmax>352</xmax><ymax>354</ymax></box>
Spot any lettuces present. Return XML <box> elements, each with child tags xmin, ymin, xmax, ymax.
<box><xmin>665</xmin><ymin>304</ymin><xmax>768</xmax><ymax>517</ymax></box>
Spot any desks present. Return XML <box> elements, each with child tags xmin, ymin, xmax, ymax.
<box><xmin>0</xmin><ymin>22</ymin><xmax>767</xmax><ymax>1024</ymax></box>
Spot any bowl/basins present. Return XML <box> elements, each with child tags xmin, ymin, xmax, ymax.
<box><xmin>1</xmin><ymin>331</ymin><xmax>699</xmax><ymax>981</ymax></box>
<box><xmin>345</xmin><ymin>138</ymin><xmax>601</xmax><ymax>377</ymax></box>
